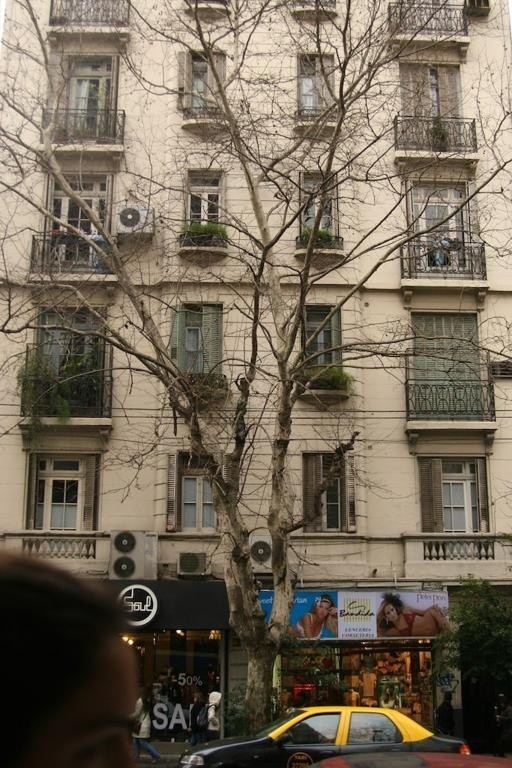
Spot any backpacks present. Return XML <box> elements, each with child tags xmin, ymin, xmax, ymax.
<box><xmin>196</xmin><ymin>702</ymin><xmax>216</xmax><ymax>729</ymax></box>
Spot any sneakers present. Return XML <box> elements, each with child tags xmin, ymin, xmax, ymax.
<box><xmin>150</xmin><ymin>754</ymin><xmax>161</xmax><ymax>764</ymax></box>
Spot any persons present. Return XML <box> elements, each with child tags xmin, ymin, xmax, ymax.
<box><xmin>0</xmin><ymin>550</ymin><xmax>163</xmax><ymax>767</ymax></box>
<box><xmin>190</xmin><ymin>691</ymin><xmax>222</xmax><ymax>747</ymax></box>
<box><xmin>344</xmin><ymin>689</ymin><xmax>360</xmax><ymax>705</ymax></box>
<box><xmin>281</xmin><ymin>689</ymin><xmax>292</xmax><ymax>709</ymax></box>
<box><xmin>377</xmin><ymin>592</ymin><xmax>449</xmax><ymax>637</ymax></box>
<box><xmin>380</xmin><ymin>684</ymin><xmax>402</xmax><ymax>707</ymax></box>
<box><xmin>289</xmin><ymin>595</ymin><xmax>337</xmax><ymax>638</ymax></box>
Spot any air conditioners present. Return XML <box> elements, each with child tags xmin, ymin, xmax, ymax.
<box><xmin>247</xmin><ymin>535</ymin><xmax>274</xmax><ymax>572</ymax></box>
<box><xmin>113</xmin><ymin>203</ymin><xmax>155</xmax><ymax>238</ymax></box>
<box><xmin>110</xmin><ymin>531</ymin><xmax>212</xmax><ymax>579</ymax></box>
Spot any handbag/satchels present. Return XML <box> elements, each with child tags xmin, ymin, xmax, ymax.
<box><xmin>132</xmin><ymin>719</ymin><xmax>142</xmax><ymax>735</ymax></box>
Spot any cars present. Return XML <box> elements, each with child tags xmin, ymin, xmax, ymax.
<box><xmin>302</xmin><ymin>751</ymin><xmax>512</xmax><ymax>768</ymax></box>
<box><xmin>178</xmin><ymin>705</ymin><xmax>471</xmax><ymax>768</ymax></box>
